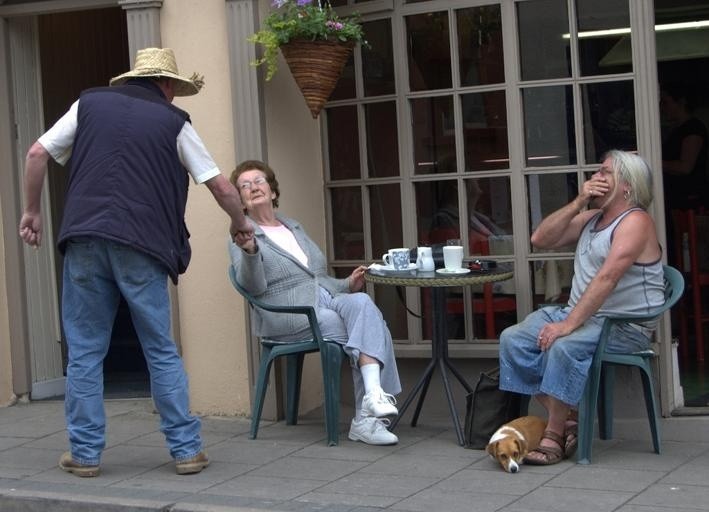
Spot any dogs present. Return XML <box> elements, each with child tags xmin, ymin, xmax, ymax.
<box><xmin>485</xmin><ymin>414</ymin><xmax>549</xmax><ymax>473</ymax></box>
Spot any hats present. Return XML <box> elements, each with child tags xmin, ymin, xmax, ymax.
<box><xmin>109</xmin><ymin>48</ymin><xmax>204</xmax><ymax>96</ymax></box>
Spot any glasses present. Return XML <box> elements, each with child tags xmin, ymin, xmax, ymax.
<box><xmin>241</xmin><ymin>178</ymin><xmax>265</xmax><ymax>188</ymax></box>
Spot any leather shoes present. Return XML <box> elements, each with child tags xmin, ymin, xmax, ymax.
<box><xmin>59</xmin><ymin>451</ymin><xmax>99</xmax><ymax>476</ymax></box>
<box><xmin>175</xmin><ymin>450</ymin><xmax>209</xmax><ymax>473</ymax></box>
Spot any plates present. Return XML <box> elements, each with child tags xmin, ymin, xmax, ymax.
<box><xmin>435</xmin><ymin>268</ymin><xmax>471</xmax><ymax>275</ymax></box>
<box><xmin>380</xmin><ymin>263</ymin><xmax>418</xmax><ymax>272</ymax></box>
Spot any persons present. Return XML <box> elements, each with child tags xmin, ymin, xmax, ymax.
<box><xmin>499</xmin><ymin>149</ymin><xmax>667</xmax><ymax>465</ymax></box>
<box><xmin>225</xmin><ymin>160</ymin><xmax>404</xmax><ymax>444</ymax></box>
<box><xmin>420</xmin><ymin>152</ymin><xmax>517</xmax><ymax>335</ymax></box>
<box><xmin>19</xmin><ymin>48</ymin><xmax>254</xmax><ymax>474</ymax></box>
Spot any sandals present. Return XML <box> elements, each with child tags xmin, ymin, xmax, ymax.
<box><xmin>523</xmin><ymin>409</ymin><xmax>577</xmax><ymax>465</ymax></box>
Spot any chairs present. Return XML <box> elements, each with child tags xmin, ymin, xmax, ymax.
<box><xmin>229</xmin><ymin>266</ymin><xmax>348</xmax><ymax>449</ymax></box>
<box><xmin>507</xmin><ymin>264</ymin><xmax>684</xmax><ymax>464</ymax></box>
<box><xmin>409</xmin><ymin>226</ymin><xmax>516</xmax><ymax>343</ymax></box>
<box><xmin>661</xmin><ymin>206</ymin><xmax>709</xmax><ymax>362</ymax></box>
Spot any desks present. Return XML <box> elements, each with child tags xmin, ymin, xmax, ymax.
<box><xmin>365</xmin><ymin>267</ymin><xmax>516</xmax><ymax>446</ymax></box>
<box><xmin>447</xmin><ymin>234</ymin><xmax>579</xmax><ymax>303</ymax></box>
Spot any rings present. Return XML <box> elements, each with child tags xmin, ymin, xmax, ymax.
<box><xmin>538</xmin><ymin>336</ymin><xmax>543</xmax><ymax>339</ymax></box>
<box><xmin>590</xmin><ymin>189</ymin><xmax>593</xmax><ymax>195</ymax></box>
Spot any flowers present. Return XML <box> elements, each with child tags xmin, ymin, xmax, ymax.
<box><xmin>244</xmin><ymin>0</ymin><xmax>370</xmax><ymax>81</ymax></box>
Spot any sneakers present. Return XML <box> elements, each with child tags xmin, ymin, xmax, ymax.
<box><xmin>348</xmin><ymin>388</ymin><xmax>399</xmax><ymax>446</ymax></box>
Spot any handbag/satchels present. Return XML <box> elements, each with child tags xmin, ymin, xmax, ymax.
<box><xmin>464</xmin><ymin>367</ymin><xmax>531</xmax><ymax>449</ymax></box>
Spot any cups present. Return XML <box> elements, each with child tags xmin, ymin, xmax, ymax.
<box><xmin>383</xmin><ymin>247</ymin><xmax>411</xmax><ymax>269</ymax></box>
<box><xmin>445</xmin><ymin>239</ymin><xmax>463</xmax><ymax>247</ymax></box>
<box><xmin>442</xmin><ymin>246</ymin><xmax>465</xmax><ymax>270</ymax></box>
<box><xmin>416</xmin><ymin>246</ymin><xmax>436</xmax><ymax>272</ymax></box>
